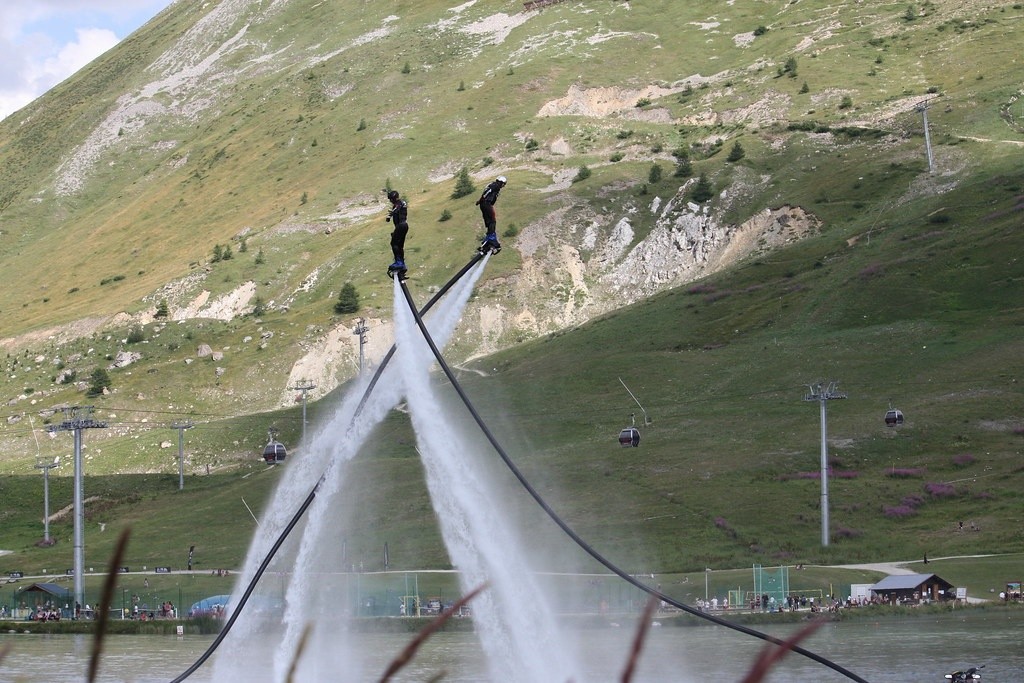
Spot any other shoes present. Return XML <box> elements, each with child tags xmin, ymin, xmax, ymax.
<box><xmin>389</xmin><ymin>262</ymin><xmax>406</xmax><ymax>268</ymax></box>
<box><xmin>485</xmin><ymin>233</ymin><xmax>496</xmax><ymax>240</ymax></box>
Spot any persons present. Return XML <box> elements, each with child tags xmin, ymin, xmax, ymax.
<box><xmin>0</xmin><ymin>590</ymin><xmax>473</xmax><ymax>620</ymax></box>
<box><xmin>438</xmin><ymin>601</ymin><xmax>444</xmax><ymax>615</ymax></box>
<box><xmin>75</xmin><ymin>601</ymin><xmax>81</xmax><ymax>620</ymax></box>
<box><xmin>959</xmin><ymin>520</ymin><xmax>963</xmax><ymax>528</ymax></box>
<box><xmin>795</xmin><ymin>564</ymin><xmax>806</xmax><ymax>570</ymax></box>
<box><xmin>428</xmin><ymin>601</ymin><xmax>432</xmax><ymax>608</ymax></box>
<box><xmin>710</xmin><ymin>596</ymin><xmax>718</xmax><ymax>610</ymax></box>
<box><xmin>476</xmin><ymin>176</ymin><xmax>508</xmax><ymax>248</ymax></box>
<box><xmin>924</xmin><ymin>552</ymin><xmax>928</xmax><ymax>565</ymax></box>
<box><xmin>723</xmin><ymin>597</ymin><xmax>728</xmax><ymax>614</ymax></box>
<box><xmin>133</xmin><ymin>603</ymin><xmax>138</xmax><ymax>619</ymax></box>
<box><xmin>750</xmin><ymin>593</ymin><xmax>755</xmax><ymax>614</ymax></box>
<box><xmin>598</xmin><ymin>587</ymin><xmax>1024</xmax><ymax>614</ymax></box>
<box><xmin>755</xmin><ymin>594</ymin><xmax>761</xmax><ymax>609</ymax></box>
<box><xmin>762</xmin><ymin>592</ymin><xmax>769</xmax><ymax>613</ymax></box>
<box><xmin>144</xmin><ymin>578</ymin><xmax>149</xmax><ymax>587</ymax></box>
<box><xmin>386</xmin><ymin>191</ymin><xmax>408</xmax><ymax>269</ymax></box>
<box><xmin>411</xmin><ymin>600</ymin><xmax>417</xmax><ymax>617</ymax></box>
<box><xmin>399</xmin><ymin>602</ymin><xmax>406</xmax><ymax>617</ymax></box>
<box><xmin>912</xmin><ymin>591</ymin><xmax>919</xmax><ymax>605</ymax></box>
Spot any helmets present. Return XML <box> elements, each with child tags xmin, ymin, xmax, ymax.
<box><xmin>388</xmin><ymin>191</ymin><xmax>399</xmax><ymax>199</ymax></box>
<box><xmin>496</xmin><ymin>176</ymin><xmax>507</xmax><ymax>185</ymax></box>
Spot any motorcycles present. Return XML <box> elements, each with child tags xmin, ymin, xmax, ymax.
<box><xmin>945</xmin><ymin>664</ymin><xmax>987</xmax><ymax>683</ymax></box>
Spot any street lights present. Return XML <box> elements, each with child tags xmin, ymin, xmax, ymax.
<box><xmin>704</xmin><ymin>568</ymin><xmax>712</xmax><ymax>601</ymax></box>
